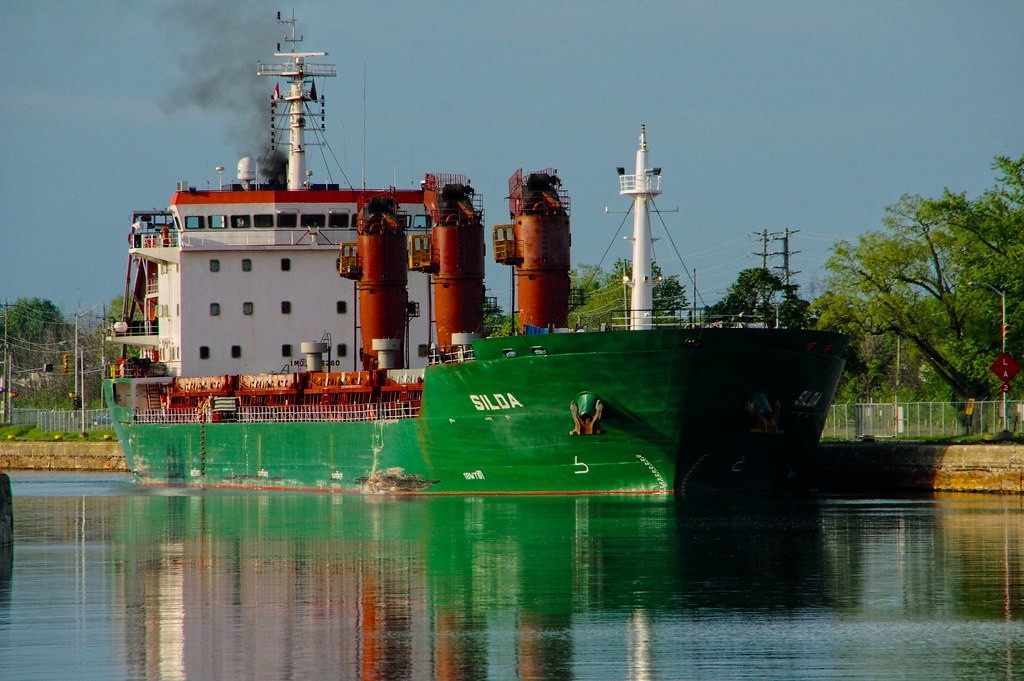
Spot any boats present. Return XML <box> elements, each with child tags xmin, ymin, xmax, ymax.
<box><xmin>102</xmin><ymin>10</ymin><xmax>853</xmax><ymax>496</ymax></box>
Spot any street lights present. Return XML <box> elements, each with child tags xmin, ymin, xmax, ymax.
<box><xmin>967</xmin><ymin>280</ymin><xmax>1008</xmax><ymax>432</ymax></box>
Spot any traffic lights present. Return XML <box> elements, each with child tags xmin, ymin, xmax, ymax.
<box><xmin>62</xmin><ymin>354</ymin><xmax>69</xmax><ymax>374</ymax></box>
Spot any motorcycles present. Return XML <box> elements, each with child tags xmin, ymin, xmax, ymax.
<box><xmin>71</xmin><ymin>396</ymin><xmax>82</xmax><ymax>410</ymax></box>
